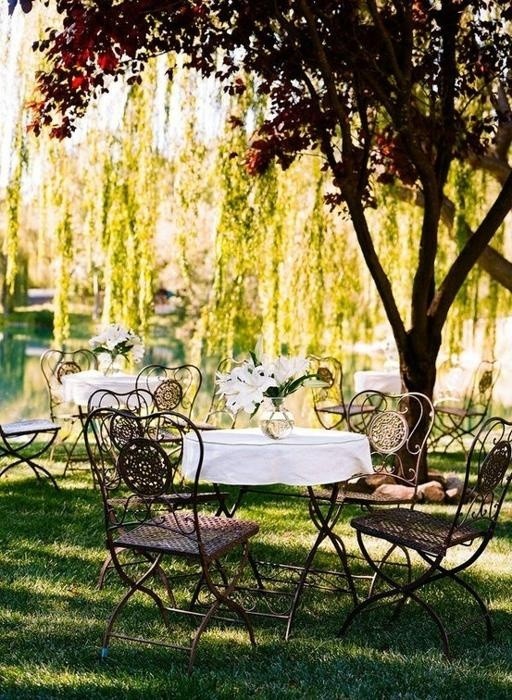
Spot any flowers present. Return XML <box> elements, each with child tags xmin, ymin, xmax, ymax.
<box><xmin>88</xmin><ymin>323</ymin><xmax>146</xmax><ymax>366</ymax></box>
